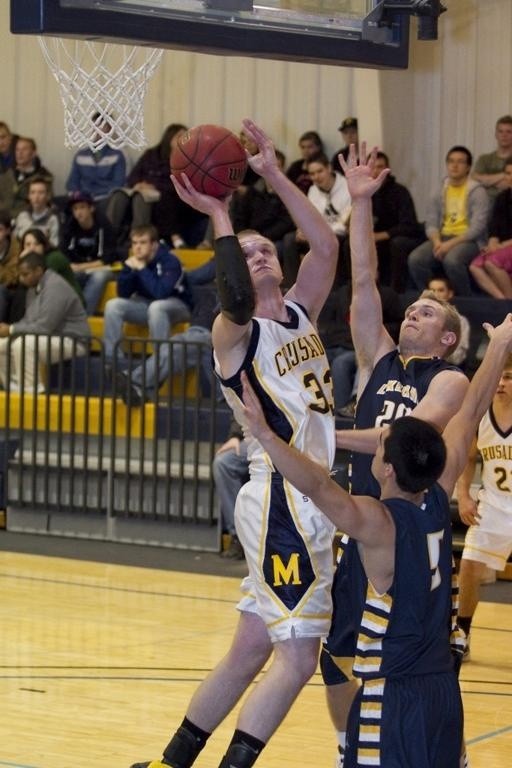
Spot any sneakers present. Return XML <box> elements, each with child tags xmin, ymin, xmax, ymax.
<box><xmin>130</xmin><ymin>758</ymin><xmax>185</xmax><ymax>768</ymax></box>
<box><xmin>98</xmin><ymin>362</ymin><xmax>149</xmax><ymax>405</ymax></box>
<box><xmin>219</xmin><ymin>539</ymin><xmax>244</xmax><ymax>560</ymax></box>
<box><xmin>450</xmin><ymin>623</ymin><xmax>472</xmax><ymax>662</ymax></box>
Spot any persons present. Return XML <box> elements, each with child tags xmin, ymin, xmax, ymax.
<box><xmin>320</xmin><ymin>140</ymin><xmax>468</xmax><ymax>768</ymax></box>
<box><xmin>240</xmin><ymin>312</ymin><xmax>512</xmax><ymax>768</ymax></box>
<box><xmin>0</xmin><ymin>113</ymin><xmax>512</xmax><ymax>561</ymax></box>
<box><xmin>130</xmin><ymin>117</ymin><xmax>340</xmax><ymax>768</ymax></box>
<box><xmin>455</xmin><ymin>357</ymin><xmax>512</xmax><ymax>665</ymax></box>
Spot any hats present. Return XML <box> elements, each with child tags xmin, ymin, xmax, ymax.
<box><xmin>337</xmin><ymin>115</ymin><xmax>357</xmax><ymax>133</ymax></box>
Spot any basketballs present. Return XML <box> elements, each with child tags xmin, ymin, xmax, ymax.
<box><xmin>170</xmin><ymin>124</ymin><xmax>248</xmax><ymax>199</ymax></box>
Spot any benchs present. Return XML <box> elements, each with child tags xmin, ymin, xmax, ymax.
<box><xmin>1</xmin><ymin>247</ymin><xmax>216</xmax><ymax>396</ymax></box>
<box><xmin>393</xmin><ymin>259</ymin><xmax>512</xmax><ymax>378</ymax></box>
